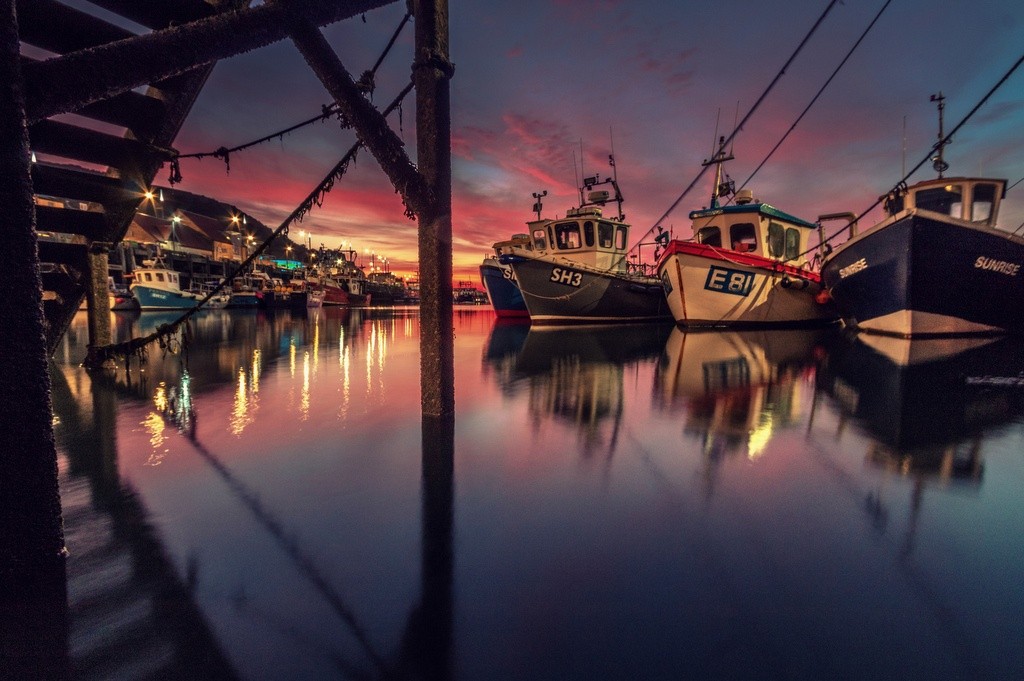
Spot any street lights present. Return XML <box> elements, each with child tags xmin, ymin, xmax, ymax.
<box><xmin>285</xmin><ymin>246</ymin><xmax>292</xmax><ymax>267</ymax></box>
<box><xmin>335</xmin><ymin>241</ymin><xmax>390</xmax><ymax>273</ymax></box>
<box><xmin>299</xmin><ymin>230</ymin><xmax>312</xmax><ymax>267</ymax></box>
<box><xmin>233</xmin><ymin>216</ymin><xmax>243</xmax><ymax>262</ymax></box>
<box><xmin>171</xmin><ymin>217</ymin><xmax>182</xmax><ymax>251</ymax></box>
<box><xmin>245</xmin><ymin>235</ymin><xmax>253</xmax><ymax>260</ymax></box>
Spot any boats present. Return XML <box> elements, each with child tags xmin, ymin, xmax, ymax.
<box><xmin>494</xmin><ymin>125</ymin><xmax>675</xmax><ymax>328</ymax></box>
<box><xmin>658</xmin><ymin>136</ymin><xmax>819</xmax><ymax>335</ymax></box>
<box><xmin>479</xmin><ymin>255</ymin><xmax>531</xmax><ymax>328</ymax></box>
<box><xmin>108</xmin><ymin>257</ymin><xmax>420</xmax><ymax>309</ymax></box>
<box><xmin>819</xmin><ymin>90</ymin><xmax>1024</xmax><ymax>379</ymax></box>
